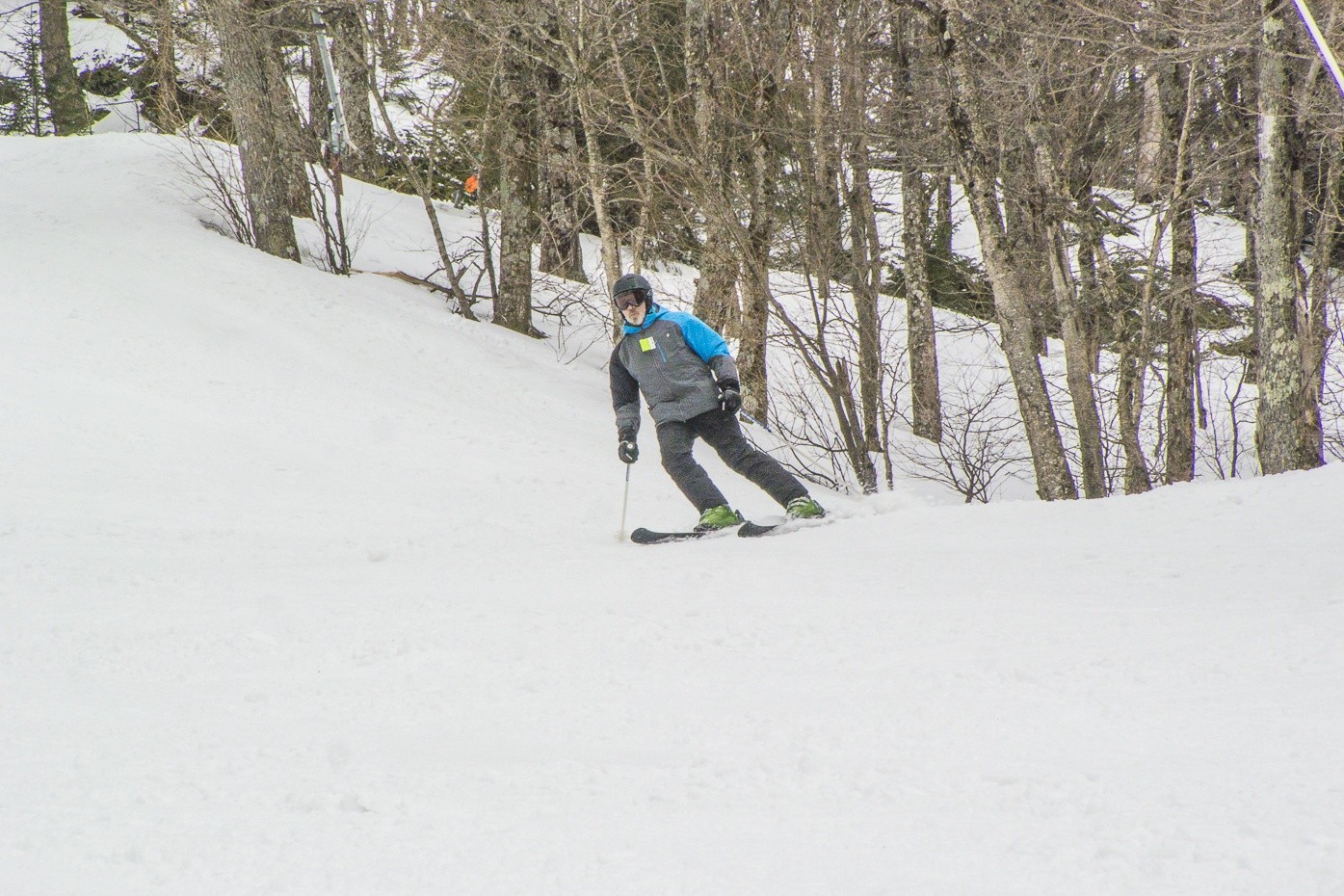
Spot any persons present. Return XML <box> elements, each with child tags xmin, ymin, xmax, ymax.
<box><xmin>460</xmin><ymin>172</ymin><xmax>478</xmax><ymax>209</ymax></box>
<box><xmin>608</xmin><ymin>274</ymin><xmax>826</xmax><ymax>533</ymax></box>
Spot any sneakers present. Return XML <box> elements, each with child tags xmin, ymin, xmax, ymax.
<box><xmin>693</xmin><ymin>504</ymin><xmax>740</xmax><ymax>531</ymax></box>
<box><xmin>785</xmin><ymin>496</ymin><xmax>822</xmax><ymax>521</ymax></box>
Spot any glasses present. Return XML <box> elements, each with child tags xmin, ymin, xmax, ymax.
<box><xmin>614</xmin><ymin>290</ymin><xmax>647</xmax><ymax>310</ymax></box>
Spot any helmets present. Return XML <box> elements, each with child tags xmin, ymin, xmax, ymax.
<box><xmin>613</xmin><ymin>274</ymin><xmax>652</xmax><ymax>319</ymax></box>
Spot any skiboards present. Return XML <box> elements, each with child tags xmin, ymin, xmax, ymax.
<box><xmin>631</xmin><ymin>522</ymin><xmax>779</xmax><ymax>545</ymax></box>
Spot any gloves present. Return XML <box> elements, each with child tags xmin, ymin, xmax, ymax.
<box><xmin>718</xmin><ymin>379</ymin><xmax>741</xmax><ymax>415</ymax></box>
<box><xmin>618</xmin><ymin>426</ymin><xmax>639</xmax><ymax>463</ymax></box>
